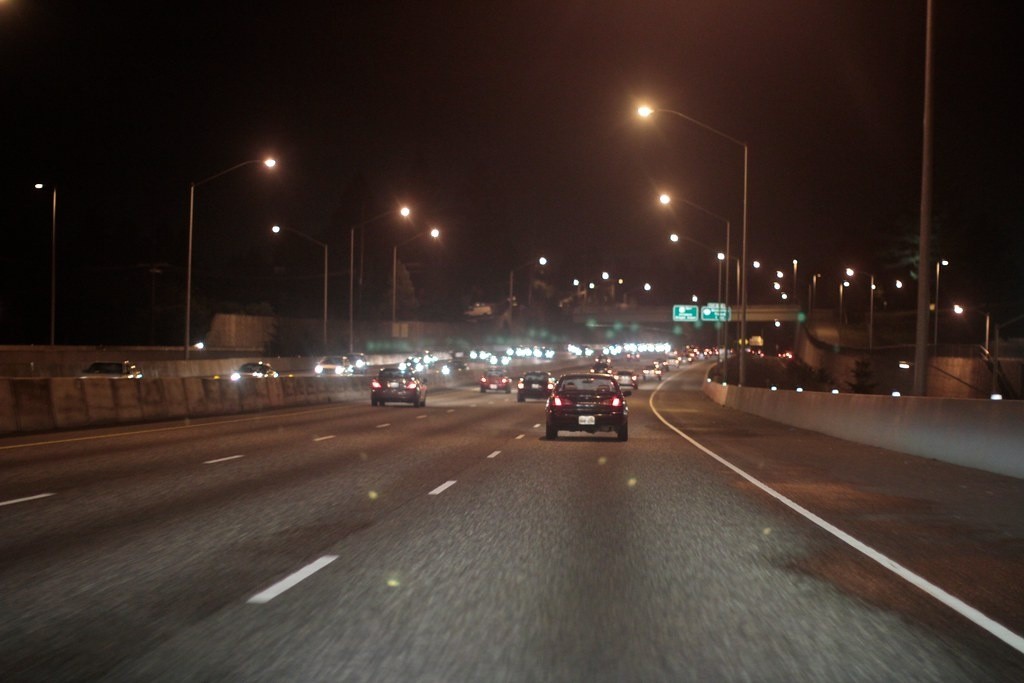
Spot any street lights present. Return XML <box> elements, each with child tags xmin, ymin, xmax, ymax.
<box><xmin>670</xmin><ymin>232</ymin><xmax>722</xmax><ymax>377</ymax></box>
<box><xmin>392</xmin><ymin>228</ymin><xmax>440</xmax><ymax>321</ymax></box>
<box><xmin>350</xmin><ymin>207</ymin><xmax>410</xmax><ymax>352</ymax></box>
<box><xmin>656</xmin><ymin>194</ymin><xmax>731</xmax><ymax>385</ymax></box>
<box><xmin>271</xmin><ymin>225</ymin><xmax>329</xmax><ymax>345</ymax></box>
<box><xmin>638</xmin><ymin>109</ymin><xmax>750</xmax><ymax>384</ymax></box>
<box><xmin>508</xmin><ymin>104</ymin><xmax>949</xmax><ymax>353</ymax></box>
<box><xmin>185</xmin><ymin>158</ymin><xmax>276</xmax><ymax>361</ymax></box>
<box><xmin>954</xmin><ymin>303</ymin><xmax>991</xmax><ymax>358</ymax></box>
<box><xmin>34</xmin><ymin>182</ymin><xmax>56</xmax><ymax>347</ymax></box>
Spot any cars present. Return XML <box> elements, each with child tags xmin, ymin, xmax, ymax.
<box><xmin>314</xmin><ymin>355</ymin><xmax>355</xmax><ymax>375</ymax></box>
<box><xmin>517</xmin><ymin>371</ymin><xmax>553</xmax><ymax>403</ymax></box>
<box><xmin>480</xmin><ymin>369</ymin><xmax>512</xmax><ymax>395</ymax></box>
<box><xmin>545</xmin><ymin>373</ymin><xmax>629</xmax><ymax>442</ymax></box>
<box><xmin>371</xmin><ymin>367</ymin><xmax>428</xmax><ymax>408</ymax></box>
<box><xmin>398</xmin><ymin>344</ymin><xmax>765</xmax><ymax>393</ymax></box>
<box><xmin>346</xmin><ymin>353</ymin><xmax>368</xmax><ymax>375</ymax></box>
<box><xmin>79</xmin><ymin>360</ymin><xmax>143</xmax><ymax>379</ymax></box>
<box><xmin>230</xmin><ymin>363</ymin><xmax>279</xmax><ymax>380</ymax></box>
